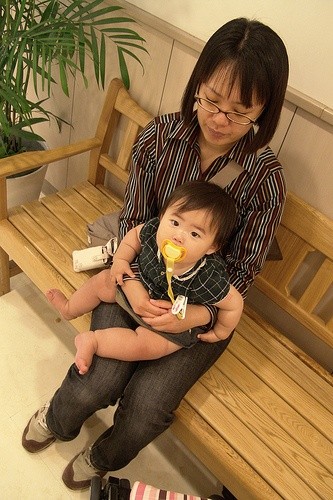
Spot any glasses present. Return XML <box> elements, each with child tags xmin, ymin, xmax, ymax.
<box><xmin>194</xmin><ymin>81</ymin><xmax>268</xmax><ymax>125</ymax></box>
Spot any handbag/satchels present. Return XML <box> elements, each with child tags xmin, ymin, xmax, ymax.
<box><xmin>87</xmin><ymin>211</ymin><xmax>120</xmax><ymax>247</ymax></box>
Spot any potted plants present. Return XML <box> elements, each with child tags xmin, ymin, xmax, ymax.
<box><xmin>0</xmin><ymin>0</ymin><xmax>151</xmax><ymax>208</ymax></box>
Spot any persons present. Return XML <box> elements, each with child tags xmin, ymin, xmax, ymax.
<box><xmin>22</xmin><ymin>17</ymin><xmax>290</xmax><ymax>492</ymax></box>
<box><xmin>48</xmin><ymin>180</ymin><xmax>244</xmax><ymax>374</ymax></box>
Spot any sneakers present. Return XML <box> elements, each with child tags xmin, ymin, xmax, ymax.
<box><xmin>62</xmin><ymin>443</ymin><xmax>107</xmax><ymax>490</ymax></box>
<box><xmin>22</xmin><ymin>398</ymin><xmax>56</xmax><ymax>452</ymax></box>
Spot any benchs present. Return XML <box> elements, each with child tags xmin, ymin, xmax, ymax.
<box><xmin>0</xmin><ymin>78</ymin><xmax>333</xmax><ymax>500</ymax></box>
